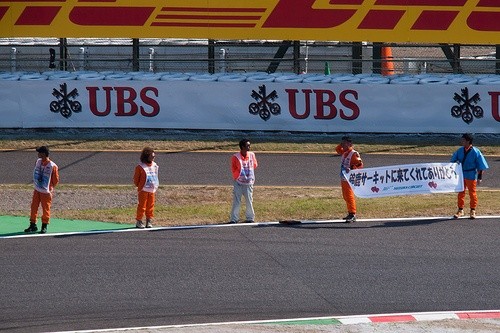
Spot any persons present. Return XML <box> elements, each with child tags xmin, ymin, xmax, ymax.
<box><xmin>24</xmin><ymin>145</ymin><xmax>60</xmax><ymax>234</ymax></box>
<box><xmin>228</xmin><ymin>139</ymin><xmax>258</xmax><ymax>224</ymax></box>
<box><xmin>336</xmin><ymin>136</ymin><xmax>363</xmax><ymax>223</ymax></box>
<box><xmin>448</xmin><ymin>133</ymin><xmax>489</xmax><ymax>219</ymax></box>
<box><xmin>132</xmin><ymin>146</ymin><xmax>160</xmax><ymax>228</ymax></box>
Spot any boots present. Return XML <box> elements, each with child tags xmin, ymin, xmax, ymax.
<box><xmin>41</xmin><ymin>223</ymin><xmax>47</xmax><ymax>233</ymax></box>
<box><xmin>136</xmin><ymin>220</ymin><xmax>145</xmax><ymax>229</ymax></box>
<box><xmin>25</xmin><ymin>223</ymin><xmax>37</xmax><ymax>232</ymax></box>
<box><xmin>146</xmin><ymin>219</ymin><xmax>153</xmax><ymax>227</ymax></box>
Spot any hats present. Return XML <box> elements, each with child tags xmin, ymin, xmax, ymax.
<box><xmin>36</xmin><ymin>146</ymin><xmax>50</xmax><ymax>153</ymax></box>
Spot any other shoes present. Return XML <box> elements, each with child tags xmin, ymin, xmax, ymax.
<box><xmin>453</xmin><ymin>210</ymin><xmax>464</xmax><ymax>219</ymax></box>
<box><xmin>343</xmin><ymin>214</ymin><xmax>356</xmax><ymax>222</ymax></box>
<box><xmin>470</xmin><ymin>210</ymin><xmax>476</xmax><ymax>219</ymax></box>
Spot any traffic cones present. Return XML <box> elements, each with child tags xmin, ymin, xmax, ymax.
<box><xmin>324</xmin><ymin>61</ymin><xmax>331</xmax><ymax>75</ymax></box>
